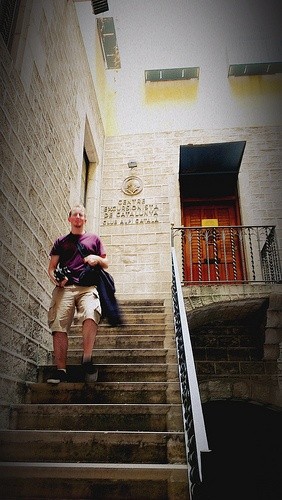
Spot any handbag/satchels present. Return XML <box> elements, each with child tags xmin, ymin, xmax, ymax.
<box><xmin>79</xmin><ymin>270</ymin><xmax>97</xmax><ymax>285</ymax></box>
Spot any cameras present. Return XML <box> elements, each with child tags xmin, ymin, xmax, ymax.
<box><xmin>54</xmin><ymin>266</ymin><xmax>73</xmax><ymax>281</ymax></box>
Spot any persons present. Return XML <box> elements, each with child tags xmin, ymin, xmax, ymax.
<box><xmin>46</xmin><ymin>205</ymin><xmax>109</xmax><ymax>384</ymax></box>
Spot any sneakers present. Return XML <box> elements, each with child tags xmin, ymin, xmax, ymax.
<box><xmin>81</xmin><ymin>356</ymin><xmax>98</xmax><ymax>382</ymax></box>
<box><xmin>47</xmin><ymin>370</ymin><xmax>68</xmax><ymax>383</ymax></box>
